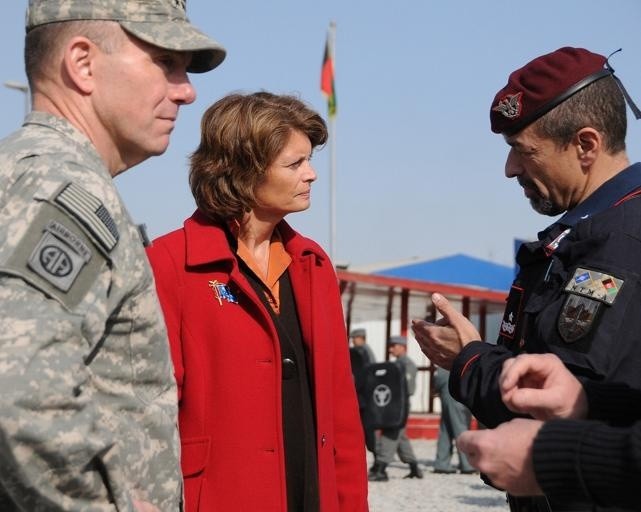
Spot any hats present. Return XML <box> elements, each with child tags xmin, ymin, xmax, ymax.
<box><xmin>25</xmin><ymin>0</ymin><xmax>227</xmax><ymax>74</ymax></box>
<box><xmin>489</xmin><ymin>46</ymin><xmax>615</xmax><ymax>136</ymax></box>
<box><xmin>349</xmin><ymin>329</ymin><xmax>365</xmax><ymax>338</ymax></box>
<box><xmin>388</xmin><ymin>337</ymin><xmax>407</xmax><ymax>345</ymax></box>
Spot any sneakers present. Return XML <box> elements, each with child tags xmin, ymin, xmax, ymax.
<box><xmin>434</xmin><ymin>463</ymin><xmax>479</xmax><ymax>474</ymax></box>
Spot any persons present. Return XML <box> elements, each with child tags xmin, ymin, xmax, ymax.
<box><xmin>454</xmin><ymin>347</ymin><xmax>641</xmax><ymax>512</ymax></box>
<box><xmin>350</xmin><ymin>325</ymin><xmax>380</xmax><ymax>471</ymax></box>
<box><xmin>0</xmin><ymin>0</ymin><xmax>225</xmax><ymax>512</ymax></box>
<box><xmin>428</xmin><ymin>361</ymin><xmax>480</xmax><ymax>476</ymax></box>
<box><xmin>363</xmin><ymin>334</ymin><xmax>425</xmax><ymax>481</ymax></box>
<box><xmin>408</xmin><ymin>43</ymin><xmax>641</xmax><ymax>511</ymax></box>
<box><xmin>140</xmin><ymin>87</ymin><xmax>372</xmax><ymax>512</ymax></box>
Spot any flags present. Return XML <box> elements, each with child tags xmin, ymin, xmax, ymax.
<box><xmin>317</xmin><ymin>28</ymin><xmax>338</xmax><ymax>122</ymax></box>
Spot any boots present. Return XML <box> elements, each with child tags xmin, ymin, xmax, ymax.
<box><xmin>403</xmin><ymin>463</ymin><xmax>423</xmax><ymax>479</ymax></box>
<box><xmin>368</xmin><ymin>461</ymin><xmax>389</xmax><ymax>481</ymax></box>
